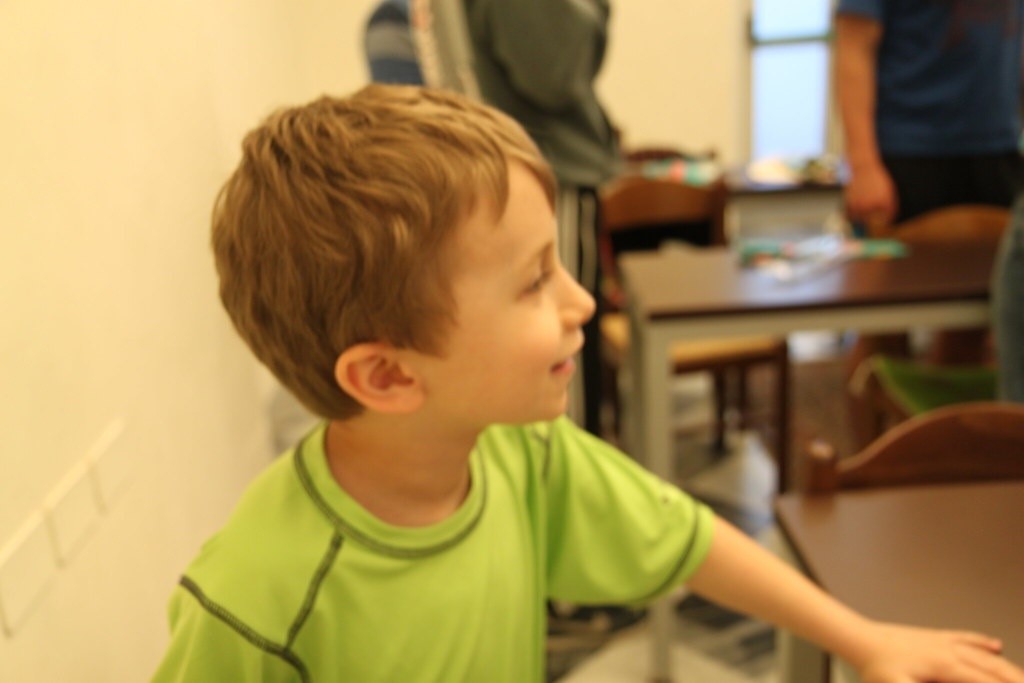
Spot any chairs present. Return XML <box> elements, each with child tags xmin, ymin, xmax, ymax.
<box><xmin>800</xmin><ymin>208</ymin><xmax>1023</xmax><ymax>492</ymax></box>
<box><xmin>593</xmin><ymin>173</ymin><xmax>794</xmax><ymax>490</ymax></box>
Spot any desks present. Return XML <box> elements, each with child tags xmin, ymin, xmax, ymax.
<box><xmin>776</xmin><ymin>477</ymin><xmax>1023</xmax><ymax>683</ymax></box>
<box><xmin>617</xmin><ymin>238</ymin><xmax>1024</xmax><ymax>683</ymax></box>
<box><xmin>576</xmin><ymin>158</ymin><xmax>848</xmax><ymax>447</ymax></box>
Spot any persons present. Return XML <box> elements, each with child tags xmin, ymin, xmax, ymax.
<box><xmin>364</xmin><ymin>0</ymin><xmax>624</xmax><ymax>632</ymax></box>
<box><xmin>151</xmin><ymin>86</ymin><xmax>1024</xmax><ymax>683</ymax></box>
<box><xmin>830</xmin><ymin>0</ymin><xmax>1024</xmax><ymax>452</ymax></box>
